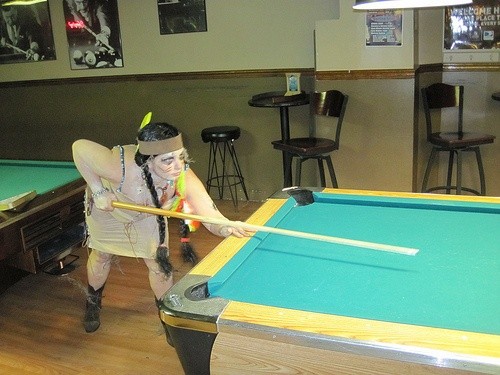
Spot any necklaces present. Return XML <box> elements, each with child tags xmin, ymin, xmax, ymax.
<box><xmin>140</xmin><ymin>167</ymin><xmax>169</xmax><ymax>192</ymax></box>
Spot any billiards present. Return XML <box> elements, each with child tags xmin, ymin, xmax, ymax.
<box><xmin>31</xmin><ymin>53</ymin><xmax>40</xmax><ymax>61</ymax></box>
<box><xmin>73</xmin><ymin>47</ymin><xmax>123</xmax><ymax>68</ymax></box>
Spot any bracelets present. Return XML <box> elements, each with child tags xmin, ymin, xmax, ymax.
<box><xmin>91</xmin><ymin>188</ymin><xmax>108</xmax><ymax>196</ymax></box>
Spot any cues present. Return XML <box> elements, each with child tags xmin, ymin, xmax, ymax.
<box><xmin>6</xmin><ymin>43</ymin><xmax>32</xmax><ymax>55</ymax></box>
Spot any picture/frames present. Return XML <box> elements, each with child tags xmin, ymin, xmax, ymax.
<box><xmin>157</xmin><ymin>0</ymin><xmax>208</xmax><ymax>35</ymax></box>
<box><xmin>63</xmin><ymin>0</ymin><xmax>124</xmax><ymax>70</ymax></box>
<box><xmin>0</xmin><ymin>0</ymin><xmax>57</xmax><ymax>65</ymax></box>
<box><xmin>365</xmin><ymin>11</ymin><xmax>403</xmax><ymax>48</ymax></box>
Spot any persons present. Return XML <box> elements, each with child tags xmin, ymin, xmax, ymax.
<box><xmin>0</xmin><ymin>0</ymin><xmax>111</xmax><ymax>61</ymax></box>
<box><xmin>72</xmin><ymin>124</ymin><xmax>254</xmax><ymax>331</ymax></box>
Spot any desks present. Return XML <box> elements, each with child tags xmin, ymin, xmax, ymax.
<box><xmin>155</xmin><ymin>186</ymin><xmax>500</xmax><ymax>375</ymax></box>
<box><xmin>0</xmin><ymin>159</ymin><xmax>88</xmax><ymax>277</ymax></box>
<box><xmin>248</xmin><ymin>91</ymin><xmax>309</xmax><ymax>187</ymax></box>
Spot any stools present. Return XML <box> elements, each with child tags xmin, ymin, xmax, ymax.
<box><xmin>271</xmin><ymin>90</ymin><xmax>349</xmax><ymax>187</ymax></box>
<box><xmin>420</xmin><ymin>83</ymin><xmax>496</xmax><ymax>195</ymax></box>
<box><xmin>201</xmin><ymin>126</ymin><xmax>248</xmax><ymax>211</ymax></box>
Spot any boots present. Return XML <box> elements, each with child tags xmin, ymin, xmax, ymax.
<box><xmin>154</xmin><ymin>295</ymin><xmax>175</xmax><ymax>347</ymax></box>
<box><xmin>84</xmin><ymin>283</ymin><xmax>105</xmax><ymax>333</ymax></box>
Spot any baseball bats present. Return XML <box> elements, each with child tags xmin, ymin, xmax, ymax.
<box><xmin>79</xmin><ymin>20</ymin><xmax>114</xmax><ymax>50</ymax></box>
<box><xmin>110</xmin><ymin>200</ymin><xmax>420</xmax><ymax>257</ymax></box>
